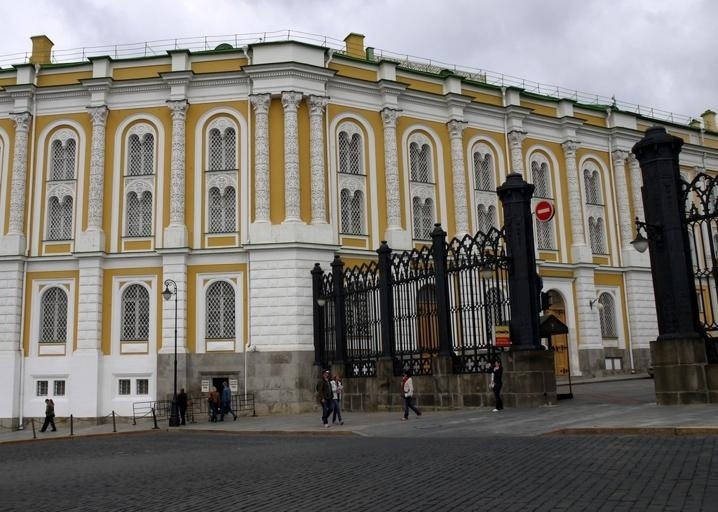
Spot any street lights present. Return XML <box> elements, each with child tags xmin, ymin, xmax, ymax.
<box><xmin>160</xmin><ymin>279</ymin><xmax>181</xmax><ymax>427</ymax></box>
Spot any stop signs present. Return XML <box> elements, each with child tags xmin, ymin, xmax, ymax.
<box><xmin>535</xmin><ymin>200</ymin><xmax>554</xmax><ymax>223</ymax></box>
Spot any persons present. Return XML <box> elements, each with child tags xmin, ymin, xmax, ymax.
<box><xmin>316</xmin><ymin>368</ymin><xmax>335</xmax><ymax>428</ymax></box>
<box><xmin>491</xmin><ymin>357</ymin><xmax>504</xmax><ymax>412</ymax></box>
<box><xmin>217</xmin><ymin>381</ymin><xmax>238</xmax><ymax>422</ymax></box>
<box><xmin>400</xmin><ymin>369</ymin><xmax>423</xmax><ymax>421</ymax></box>
<box><xmin>209</xmin><ymin>386</ymin><xmax>220</xmax><ymax>422</ymax></box>
<box><xmin>176</xmin><ymin>388</ymin><xmax>188</xmax><ymax>426</ymax></box>
<box><xmin>207</xmin><ymin>392</ymin><xmax>214</xmax><ymax>422</ymax></box>
<box><xmin>330</xmin><ymin>372</ymin><xmax>344</xmax><ymax>426</ymax></box>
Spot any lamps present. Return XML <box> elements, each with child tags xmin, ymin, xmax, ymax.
<box><xmin>629</xmin><ymin>215</ymin><xmax>668</xmax><ymax>255</ymax></box>
<box><xmin>314</xmin><ymin>285</ymin><xmax>337</xmax><ymax>307</ymax></box>
